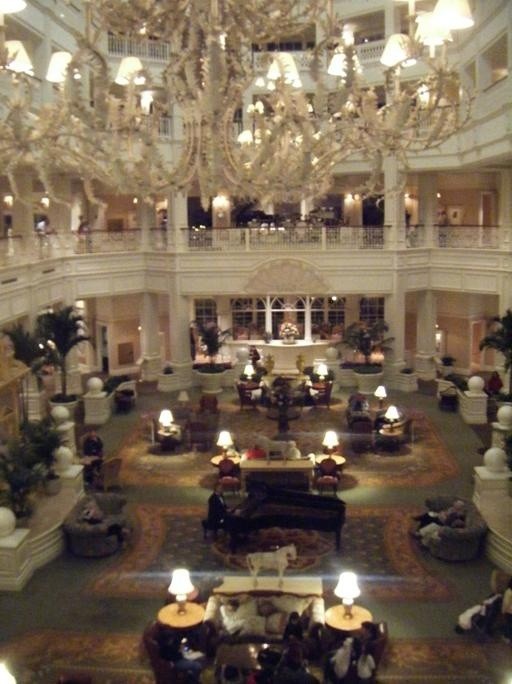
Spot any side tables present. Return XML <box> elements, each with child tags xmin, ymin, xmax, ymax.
<box><xmin>80</xmin><ymin>456</ymin><xmax>100</xmax><ymax>483</ymax></box>
<box><xmin>209</xmin><ymin>454</ymin><xmax>243</xmax><ymax>476</ymax></box>
<box><xmin>315</xmin><ymin>453</ymin><xmax>346</xmax><ymax>477</ymax></box>
<box><xmin>379</xmin><ymin>428</ymin><xmax>403</xmax><ymax>451</ymax></box>
<box><xmin>326</xmin><ymin>604</ymin><xmax>373</xmax><ymax>630</ymax></box>
<box><xmin>373</xmin><ymin>404</ymin><xmax>387</xmax><ymax>419</ymax></box>
<box><xmin>157</xmin><ymin>601</ymin><xmax>205</xmax><ymax>628</ymax></box>
<box><xmin>158</xmin><ymin>427</ymin><xmax>178</xmax><ymax>451</ymax></box>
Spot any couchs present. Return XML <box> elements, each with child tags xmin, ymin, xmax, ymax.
<box><xmin>195</xmin><ymin>395</ymin><xmax>220</xmax><ymax>423</ymax></box>
<box><xmin>346</xmin><ymin>393</ymin><xmax>372</xmax><ymax>427</ymax></box>
<box><xmin>151</xmin><ymin>407</ymin><xmax>191</xmax><ymax>447</ymax></box>
<box><xmin>329</xmin><ymin>621</ymin><xmax>388</xmax><ymax>676</ymax></box>
<box><xmin>411</xmin><ymin>494</ymin><xmax>486</xmax><ymax>561</ymax></box>
<box><xmin>142</xmin><ymin>620</ymin><xmax>202</xmax><ymax>684</ymax></box>
<box><xmin>186</xmin><ymin>420</ymin><xmax>214</xmax><ymax>451</ymax></box>
<box><xmin>204</xmin><ymin>594</ymin><xmax>327</xmax><ymax>659</ymax></box>
<box><xmin>352</xmin><ymin>421</ymin><xmax>375</xmax><ymax>454</ymax></box>
<box><xmin>374</xmin><ymin>402</ymin><xmax>411</xmax><ymax>444</ymax></box>
<box><xmin>62</xmin><ymin>490</ymin><xmax>128</xmax><ymax>558</ymax></box>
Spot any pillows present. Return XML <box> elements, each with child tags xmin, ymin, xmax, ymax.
<box><xmin>220</xmin><ymin>601</ymin><xmax>258</xmax><ymax>634</ymax></box>
<box><xmin>238</xmin><ymin>615</ymin><xmax>265</xmax><ymax>641</ymax></box>
<box><xmin>266</xmin><ymin>611</ymin><xmax>287</xmax><ymax>634</ymax></box>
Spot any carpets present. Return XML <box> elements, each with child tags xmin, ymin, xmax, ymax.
<box><xmin>113</xmin><ymin>397</ymin><xmax>461</xmax><ymax>488</ymax></box>
<box><xmin>0</xmin><ymin>631</ymin><xmax>512</xmax><ymax>684</ymax></box>
<box><xmin>84</xmin><ymin>502</ymin><xmax>456</xmax><ymax>602</ymax></box>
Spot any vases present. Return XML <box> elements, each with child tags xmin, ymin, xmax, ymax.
<box><xmin>283</xmin><ymin>336</ymin><xmax>293</xmax><ymax>343</ymax></box>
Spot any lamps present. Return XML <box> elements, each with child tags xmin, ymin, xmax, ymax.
<box><xmin>333</xmin><ymin>572</ymin><xmax>362</xmax><ymax>620</ymax></box>
<box><xmin>0</xmin><ymin>0</ymin><xmax>475</xmax><ymax>229</ymax></box>
<box><xmin>321</xmin><ymin>430</ymin><xmax>339</xmax><ymax>459</ymax></box>
<box><xmin>158</xmin><ymin>409</ymin><xmax>174</xmax><ymax>432</ymax></box>
<box><xmin>216</xmin><ymin>431</ymin><xmax>234</xmax><ymax>460</ymax></box>
<box><xmin>176</xmin><ymin>390</ymin><xmax>190</xmax><ymax>408</ymax></box>
<box><xmin>374</xmin><ymin>385</ymin><xmax>387</xmax><ymax>409</ymax></box>
<box><xmin>316</xmin><ymin>364</ymin><xmax>328</xmax><ymax>386</ymax></box>
<box><xmin>167</xmin><ymin>568</ymin><xmax>195</xmax><ymax>616</ymax></box>
<box><xmin>244</xmin><ymin>365</ymin><xmax>256</xmax><ymax>385</ymax></box>
<box><xmin>385</xmin><ymin>405</ymin><xmax>399</xmax><ymax>433</ymax></box>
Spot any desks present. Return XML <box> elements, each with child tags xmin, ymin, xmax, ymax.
<box><xmin>240</xmin><ymin>459</ymin><xmax>314</xmax><ymax>501</ymax></box>
<box><xmin>213</xmin><ymin>575</ymin><xmax>324</xmax><ymax>597</ymax></box>
<box><xmin>267</xmin><ymin>378</ymin><xmax>304</xmax><ymax>421</ymax></box>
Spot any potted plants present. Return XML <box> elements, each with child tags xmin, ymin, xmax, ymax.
<box><xmin>345</xmin><ymin>320</ymin><xmax>394</xmax><ymax>396</ymax></box>
<box><xmin>0</xmin><ymin>301</ymin><xmax>94</xmax><ymax>422</ymax></box>
<box><xmin>439</xmin><ymin>356</ymin><xmax>457</xmax><ymax>378</ymax></box>
<box><xmin>192</xmin><ymin>318</ymin><xmax>238</xmax><ymax>395</ymax></box>
<box><xmin>20</xmin><ymin>416</ymin><xmax>75</xmax><ymax>496</ymax></box>
<box><xmin>393</xmin><ymin>367</ymin><xmax>419</xmax><ymax>392</ymax></box>
<box><xmin>157</xmin><ymin>363</ymin><xmax>179</xmax><ymax>392</ymax></box>
<box><xmin>0</xmin><ymin>435</ymin><xmax>47</xmax><ymax>529</ymax></box>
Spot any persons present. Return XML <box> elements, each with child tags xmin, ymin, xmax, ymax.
<box><xmin>453</xmin><ymin>569</ymin><xmax>509</xmax><ymax>640</ymax></box>
<box><xmin>30</xmin><ymin>215</ymin><xmax>54</xmax><ymax>258</ymax></box>
<box><xmin>246</xmin><ymin>669</ymin><xmax>269</xmax><ymax>683</ymax></box>
<box><xmin>158</xmin><ymin>213</ymin><xmax>172</xmax><ymax>249</ymax></box>
<box><xmin>77</xmin><ymin>214</ymin><xmax>94</xmax><ymax>247</ymax></box>
<box><xmin>498</xmin><ymin>568</ymin><xmax>512</xmax><ymax>645</ymax></box>
<box><xmin>274</xmin><ymin>636</ymin><xmax>319</xmax><ymax>684</ymax></box>
<box><xmin>83</xmin><ymin>514</ymin><xmax>133</xmax><ymax>551</ymax></box>
<box><xmin>412</xmin><ymin>500</ymin><xmax>465</xmax><ymax>539</ymax></box>
<box><xmin>282</xmin><ymin>612</ymin><xmax>304</xmax><ymax>643</ymax></box>
<box><xmin>272</xmin><ymin>391</ymin><xmax>290</xmax><ymax>434</ymax></box>
<box><xmin>488</xmin><ymin>370</ymin><xmax>504</xmax><ymax>394</ymax></box>
<box><xmin>152</xmin><ymin>622</ymin><xmax>208</xmax><ymax>682</ymax></box>
<box><xmin>403</xmin><ymin>207</ymin><xmax>414</xmax><ymax>242</ymax></box>
<box><xmin>249</xmin><ymin>345</ymin><xmax>260</xmax><ymax>367</ymax></box>
<box><xmin>83</xmin><ymin>428</ymin><xmax>104</xmax><ymax>475</ymax></box>
<box><xmin>217</xmin><ymin>662</ymin><xmax>243</xmax><ymax>684</ymax></box>
<box><xmin>219</xmin><ymin>599</ymin><xmax>253</xmax><ymax>637</ymax></box>
<box><xmin>207</xmin><ymin>482</ymin><xmax>248</xmax><ymax>542</ymax></box>
<box><xmin>327</xmin><ymin>621</ymin><xmax>379</xmax><ymax>684</ymax></box>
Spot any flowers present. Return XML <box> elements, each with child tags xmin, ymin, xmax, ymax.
<box><xmin>278</xmin><ymin>320</ymin><xmax>298</xmax><ymax>338</ymax></box>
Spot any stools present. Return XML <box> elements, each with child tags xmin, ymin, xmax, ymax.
<box><xmin>202</xmin><ymin>519</ymin><xmax>227</xmax><ymax>539</ymax></box>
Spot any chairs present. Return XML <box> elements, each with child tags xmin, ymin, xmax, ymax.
<box><xmin>215</xmin><ymin>459</ymin><xmax>241</xmax><ymax>495</ymax></box>
<box><xmin>237</xmin><ymin>383</ymin><xmax>256</xmax><ymax>411</ymax></box>
<box><xmin>315</xmin><ymin>458</ymin><xmax>341</xmax><ymax>498</ymax></box>
<box><xmin>91</xmin><ymin>456</ymin><xmax>123</xmax><ymax>492</ymax></box>
<box><xmin>314</xmin><ymin>381</ymin><xmax>333</xmax><ymax>410</ymax></box>
<box><xmin>79</xmin><ymin>431</ymin><xmax>98</xmax><ymax>457</ymax></box>
